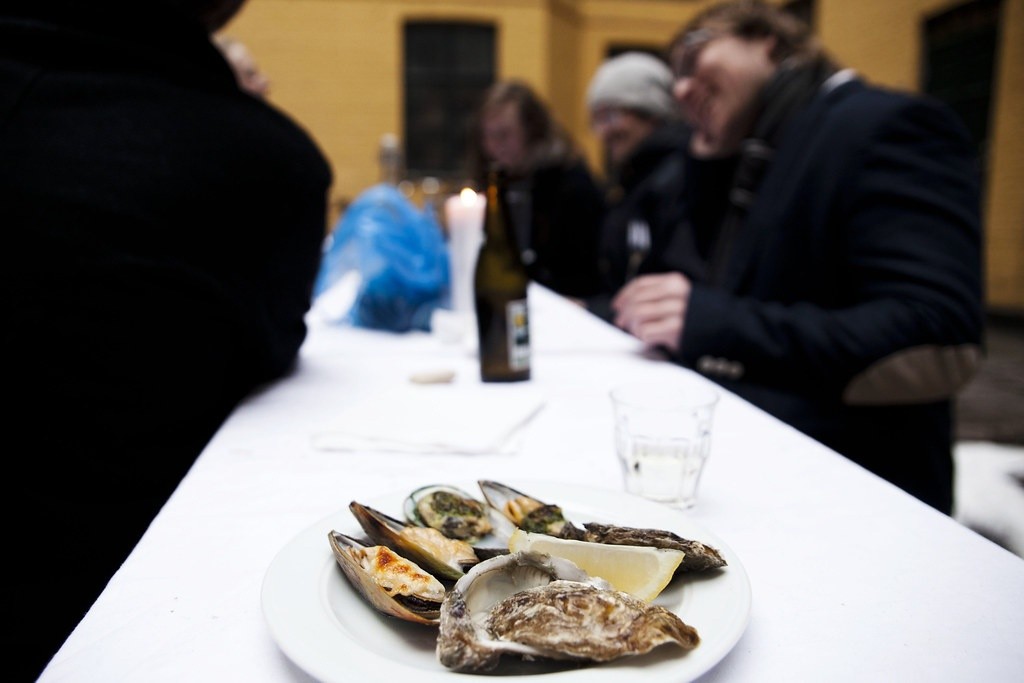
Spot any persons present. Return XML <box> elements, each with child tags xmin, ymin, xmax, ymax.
<box><xmin>0</xmin><ymin>0</ymin><xmax>338</xmax><ymax>683</ymax></box>
<box><xmin>480</xmin><ymin>1</ymin><xmax>988</xmax><ymax>518</ymax></box>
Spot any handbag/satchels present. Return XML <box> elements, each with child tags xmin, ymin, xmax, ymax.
<box><xmin>311</xmin><ymin>183</ymin><xmax>452</xmax><ymax>334</ymax></box>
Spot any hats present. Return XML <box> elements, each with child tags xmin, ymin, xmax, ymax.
<box><xmin>587</xmin><ymin>53</ymin><xmax>686</xmax><ymax>126</ymax></box>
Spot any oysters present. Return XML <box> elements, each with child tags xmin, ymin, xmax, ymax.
<box><xmin>348</xmin><ymin>500</ymin><xmax>482</xmax><ymax>583</ymax></box>
<box><xmin>328</xmin><ymin>531</ymin><xmax>445</xmax><ymax>624</ymax></box>
<box><xmin>436</xmin><ymin>550</ymin><xmax>700</xmax><ymax>676</ymax></box>
<box><xmin>404</xmin><ymin>485</ymin><xmax>515</xmax><ymax>562</ymax></box>
<box><xmin>479</xmin><ymin>480</ymin><xmax>727</xmax><ymax>573</ymax></box>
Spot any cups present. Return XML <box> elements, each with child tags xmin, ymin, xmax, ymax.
<box><xmin>608</xmin><ymin>376</ymin><xmax>721</xmax><ymax>509</ymax></box>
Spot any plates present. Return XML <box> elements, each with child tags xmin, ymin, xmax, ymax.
<box><xmin>258</xmin><ymin>490</ymin><xmax>752</xmax><ymax>683</ymax></box>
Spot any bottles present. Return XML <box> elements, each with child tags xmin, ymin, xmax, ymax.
<box><xmin>472</xmin><ymin>161</ymin><xmax>532</xmax><ymax>382</ymax></box>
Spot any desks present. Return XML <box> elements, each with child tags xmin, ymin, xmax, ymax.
<box><xmin>33</xmin><ymin>253</ymin><xmax>1024</xmax><ymax>683</ymax></box>
